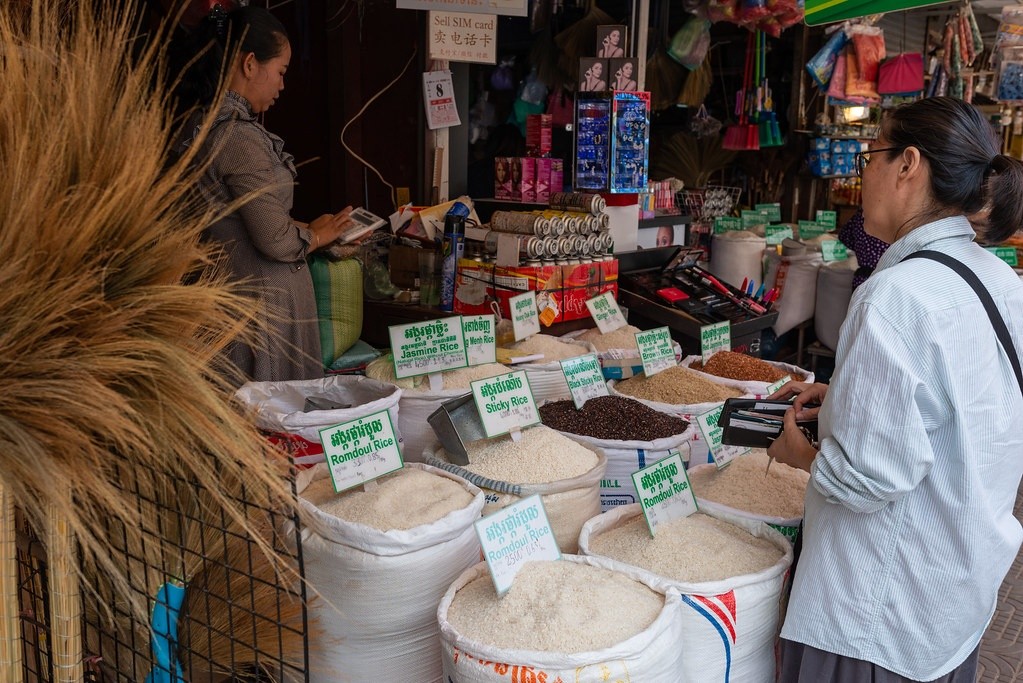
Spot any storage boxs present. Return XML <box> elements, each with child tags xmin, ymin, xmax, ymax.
<box><xmin>453</xmin><ymin>259</ymin><xmax>618</xmax><ymax>325</ymax></box>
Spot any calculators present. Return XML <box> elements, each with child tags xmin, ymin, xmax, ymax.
<box><xmin>334</xmin><ymin>206</ymin><xmax>387</xmax><ymax>245</ymax></box>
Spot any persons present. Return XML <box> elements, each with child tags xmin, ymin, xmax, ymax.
<box><xmin>655</xmin><ymin>225</ymin><xmax>675</xmax><ymax>249</ymax></box>
<box><xmin>767</xmin><ymin>95</ymin><xmax>1023</xmax><ymax>683</ymax></box>
<box><xmin>495</xmin><ymin>157</ymin><xmax>510</xmax><ymax>184</ymax></box>
<box><xmin>183</xmin><ymin>17</ymin><xmax>353</xmax><ymax>391</ymax></box>
<box><xmin>599</xmin><ymin>29</ymin><xmax>623</xmax><ymax>59</ymax></box>
<box><xmin>581</xmin><ymin>61</ymin><xmax>606</xmax><ymax>92</ymax></box>
<box><xmin>610</xmin><ymin>62</ymin><xmax>636</xmax><ymax>92</ymax></box>
<box><xmin>511</xmin><ymin>157</ymin><xmax>522</xmax><ymax>184</ymax></box>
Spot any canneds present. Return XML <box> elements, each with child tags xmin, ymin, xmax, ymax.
<box><xmin>483</xmin><ymin>190</ymin><xmax>613</xmax><ymax>259</ymax></box>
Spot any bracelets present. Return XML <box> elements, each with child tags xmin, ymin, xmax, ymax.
<box><xmin>307</xmin><ymin>226</ymin><xmax>321</xmax><ymax>249</ymax></box>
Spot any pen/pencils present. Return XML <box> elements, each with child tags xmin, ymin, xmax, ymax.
<box><xmin>684</xmin><ymin>265</ymin><xmax>781</xmax><ymax>316</ymax></box>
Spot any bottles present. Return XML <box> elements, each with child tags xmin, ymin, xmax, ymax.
<box><xmin>417</xmin><ymin>189</ymin><xmax>614</xmax><ymax>314</ymax></box>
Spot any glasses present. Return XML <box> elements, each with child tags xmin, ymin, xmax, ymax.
<box><xmin>854</xmin><ymin>147</ymin><xmax>908</xmax><ymax>179</ymax></box>
